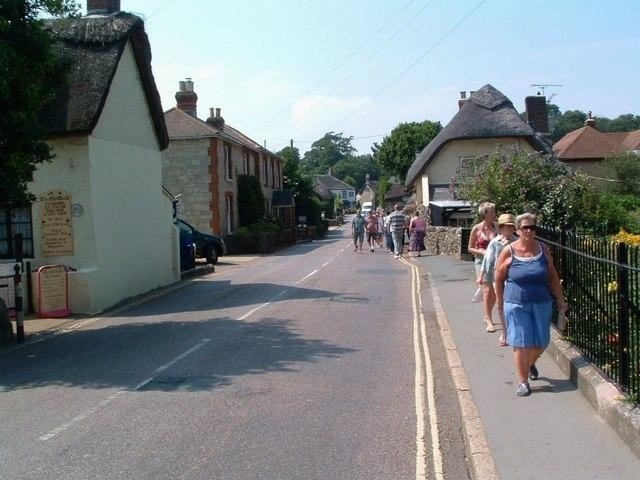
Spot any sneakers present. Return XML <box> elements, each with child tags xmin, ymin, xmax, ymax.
<box><xmin>386</xmin><ymin>247</ymin><xmax>402</xmax><ymax>259</ymax></box>
<box><xmin>528</xmin><ymin>364</ymin><xmax>538</xmax><ymax>380</ymax></box>
<box><xmin>517</xmin><ymin>383</ymin><xmax>531</xmax><ymax>396</ymax></box>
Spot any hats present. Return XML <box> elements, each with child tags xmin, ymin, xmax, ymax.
<box><xmin>498</xmin><ymin>214</ymin><xmax>515</xmax><ymax>230</ymax></box>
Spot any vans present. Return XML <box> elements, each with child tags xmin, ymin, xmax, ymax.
<box><xmin>360</xmin><ymin>202</ymin><xmax>373</xmax><ymax>216</ymax></box>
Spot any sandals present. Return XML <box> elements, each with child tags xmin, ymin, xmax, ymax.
<box><xmin>484</xmin><ymin>316</ymin><xmax>495</xmax><ymax>332</ymax></box>
<box><xmin>498</xmin><ymin>333</ymin><xmax>509</xmax><ymax>346</ymax></box>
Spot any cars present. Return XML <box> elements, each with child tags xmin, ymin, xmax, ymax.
<box><xmin>173</xmin><ymin>218</ymin><xmax>227</xmax><ymax>265</ymax></box>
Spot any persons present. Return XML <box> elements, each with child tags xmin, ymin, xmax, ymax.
<box><xmin>472</xmin><ymin>214</ymin><xmax>519</xmax><ymax>344</ymax></box>
<box><xmin>495</xmin><ymin>213</ymin><xmax>568</xmax><ymax>398</ymax></box>
<box><xmin>467</xmin><ymin>201</ymin><xmax>498</xmax><ymax>333</ymax></box>
<box><xmin>349</xmin><ymin>205</ymin><xmax>428</xmax><ymax>257</ymax></box>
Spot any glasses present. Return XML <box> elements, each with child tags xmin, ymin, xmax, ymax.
<box><xmin>518</xmin><ymin>224</ymin><xmax>536</xmax><ymax>232</ymax></box>
